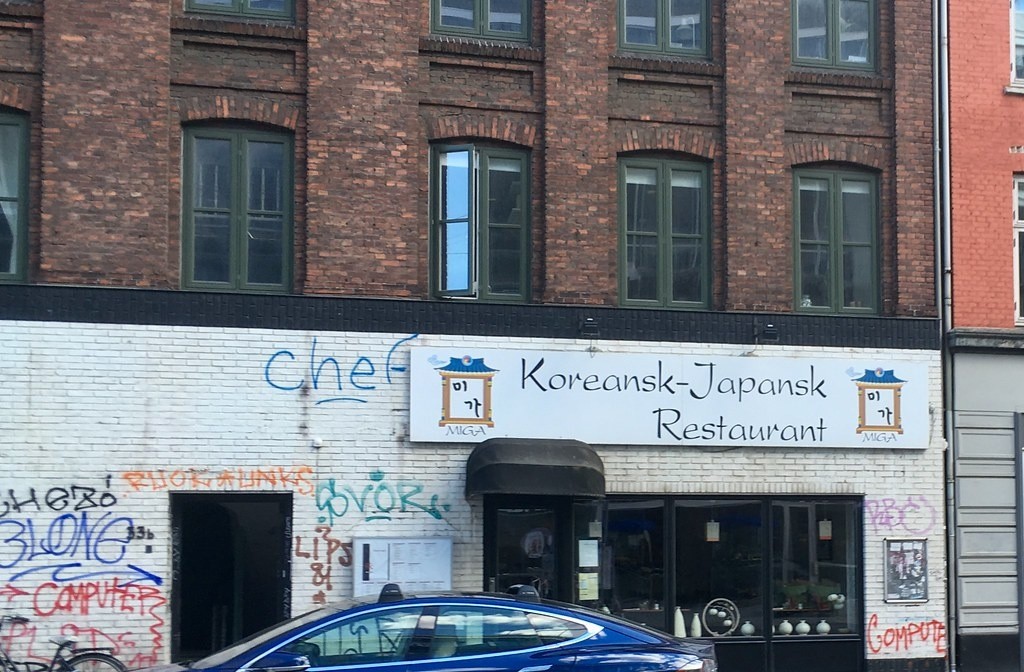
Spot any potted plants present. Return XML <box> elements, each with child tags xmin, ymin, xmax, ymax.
<box><xmin>782</xmin><ymin>581</ymin><xmax>841</xmax><ymax>611</ymax></box>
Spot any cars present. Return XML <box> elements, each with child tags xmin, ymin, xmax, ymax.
<box><xmin>127</xmin><ymin>588</ymin><xmax>720</xmax><ymax>672</ymax></box>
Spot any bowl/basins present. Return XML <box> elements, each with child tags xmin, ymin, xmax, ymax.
<box><xmin>838</xmin><ymin>628</ymin><xmax>850</xmax><ymax>634</ymax></box>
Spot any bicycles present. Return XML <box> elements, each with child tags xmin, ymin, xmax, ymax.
<box><xmin>0</xmin><ymin>614</ymin><xmax>128</xmax><ymax>672</ymax></box>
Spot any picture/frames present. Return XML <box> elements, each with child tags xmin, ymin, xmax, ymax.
<box><xmin>883</xmin><ymin>537</ymin><xmax>929</xmax><ymax>603</ymax></box>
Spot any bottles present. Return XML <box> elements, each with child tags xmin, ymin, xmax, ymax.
<box><xmin>740</xmin><ymin>620</ymin><xmax>754</xmax><ymax>636</ymax></box>
<box><xmin>778</xmin><ymin>619</ymin><xmax>793</xmax><ymax>635</ymax></box>
<box><xmin>816</xmin><ymin>620</ymin><xmax>831</xmax><ymax>635</ymax></box>
<box><xmin>674</xmin><ymin>606</ymin><xmax>686</xmax><ymax>638</ymax></box>
<box><xmin>690</xmin><ymin>613</ymin><xmax>701</xmax><ymax>637</ymax></box>
